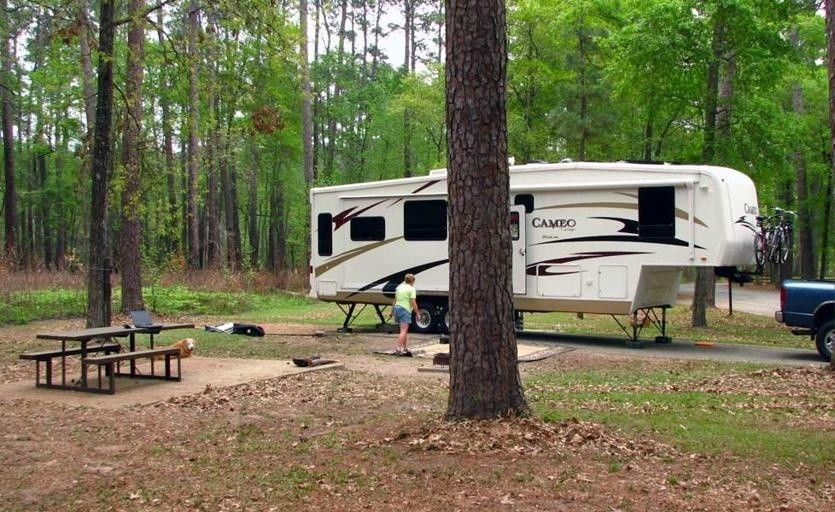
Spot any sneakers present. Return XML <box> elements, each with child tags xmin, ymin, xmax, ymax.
<box><xmin>395</xmin><ymin>349</ymin><xmax>401</xmax><ymax>355</ymax></box>
<box><xmin>403</xmin><ymin>348</ymin><xmax>411</xmax><ymax>354</ymax></box>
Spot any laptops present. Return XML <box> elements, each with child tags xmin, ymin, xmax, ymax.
<box><xmin>130</xmin><ymin>310</ymin><xmax>164</xmax><ymax>328</ymax></box>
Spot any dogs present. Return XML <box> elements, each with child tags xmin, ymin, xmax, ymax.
<box><xmin>148</xmin><ymin>337</ymin><xmax>194</xmax><ymax>362</ymax></box>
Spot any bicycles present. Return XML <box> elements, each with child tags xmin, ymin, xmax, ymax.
<box><xmin>752</xmin><ymin>207</ymin><xmax>794</xmax><ymax>267</ymax></box>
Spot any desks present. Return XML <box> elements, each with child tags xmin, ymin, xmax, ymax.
<box><xmin>36</xmin><ymin>323</ymin><xmax>195</xmax><ymax>394</ymax></box>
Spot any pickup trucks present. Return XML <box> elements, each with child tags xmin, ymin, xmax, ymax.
<box><xmin>775</xmin><ymin>280</ymin><xmax>835</xmax><ymax>360</ymax></box>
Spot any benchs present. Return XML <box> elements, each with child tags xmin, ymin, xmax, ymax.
<box><xmin>20</xmin><ymin>344</ymin><xmax>120</xmax><ymax>387</ymax></box>
<box><xmin>82</xmin><ymin>347</ymin><xmax>181</xmax><ymax>394</ymax></box>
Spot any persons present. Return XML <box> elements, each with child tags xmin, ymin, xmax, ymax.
<box><xmin>389</xmin><ymin>273</ymin><xmax>421</xmax><ymax>356</ymax></box>
